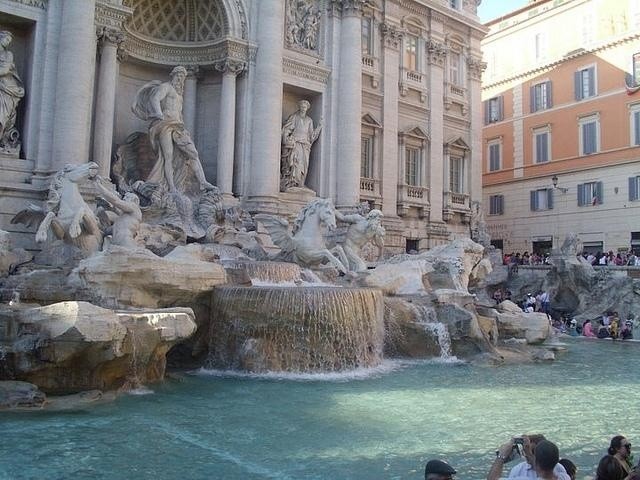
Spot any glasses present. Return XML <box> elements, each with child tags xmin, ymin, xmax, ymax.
<box><xmin>623</xmin><ymin>444</ymin><xmax>632</xmax><ymax>449</ymax></box>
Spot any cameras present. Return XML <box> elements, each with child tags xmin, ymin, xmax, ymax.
<box><xmin>513</xmin><ymin>437</ymin><xmax>524</xmax><ymax>446</ymax></box>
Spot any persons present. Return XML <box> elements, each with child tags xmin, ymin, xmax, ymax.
<box><xmin>0</xmin><ymin>31</ymin><xmax>26</xmax><ymax>154</ymax></box>
<box><xmin>279</xmin><ymin>99</ymin><xmax>324</xmax><ymax>193</ymax></box>
<box><xmin>89</xmin><ymin>174</ymin><xmax>164</xmax><ymax>261</ymax></box>
<box><xmin>595</xmin><ymin>436</ymin><xmax>639</xmax><ymax>480</ymax></box>
<box><xmin>501</xmin><ymin>249</ymin><xmax>640</xmax><ymax>266</ymax></box>
<box><xmin>492</xmin><ymin>286</ymin><xmax>636</xmax><ymax>340</ymax></box>
<box><xmin>130</xmin><ymin>66</ymin><xmax>218</xmax><ymax>193</ymax></box>
<box><xmin>335</xmin><ymin>208</ymin><xmax>386</xmax><ymax>273</ymax></box>
<box><xmin>425</xmin><ymin>460</ymin><xmax>457</xmax><ymax>480</ymax></box>
<box><xmin>284</xmin><ymin>0</ymin><xmax>322</xmax><ymax>51</ymax></box>
<box><xmin>486</xmin><ymin>433</ymin><xmax>578</xmax><ymax>480</ymax></box>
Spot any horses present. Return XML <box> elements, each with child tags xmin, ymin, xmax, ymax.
<box><xmin>33</xmin><ymin>162</ymin><xmax>101</xmax><ymax>259</ymax></box>
<box><xmin>273</xmin><ymin>198</ymin><xmax>349</xmax><ymax>275</ymax></box>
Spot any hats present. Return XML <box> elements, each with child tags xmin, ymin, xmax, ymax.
<box><xmin>427</xmin><ymin>459</ymin><xmax>456</xmax><ymax>474</ymax></box>
<box><xmin>571</xmin><ymin>319</ymin><xmax>577</xmax><ymax>324</ymax></box>
